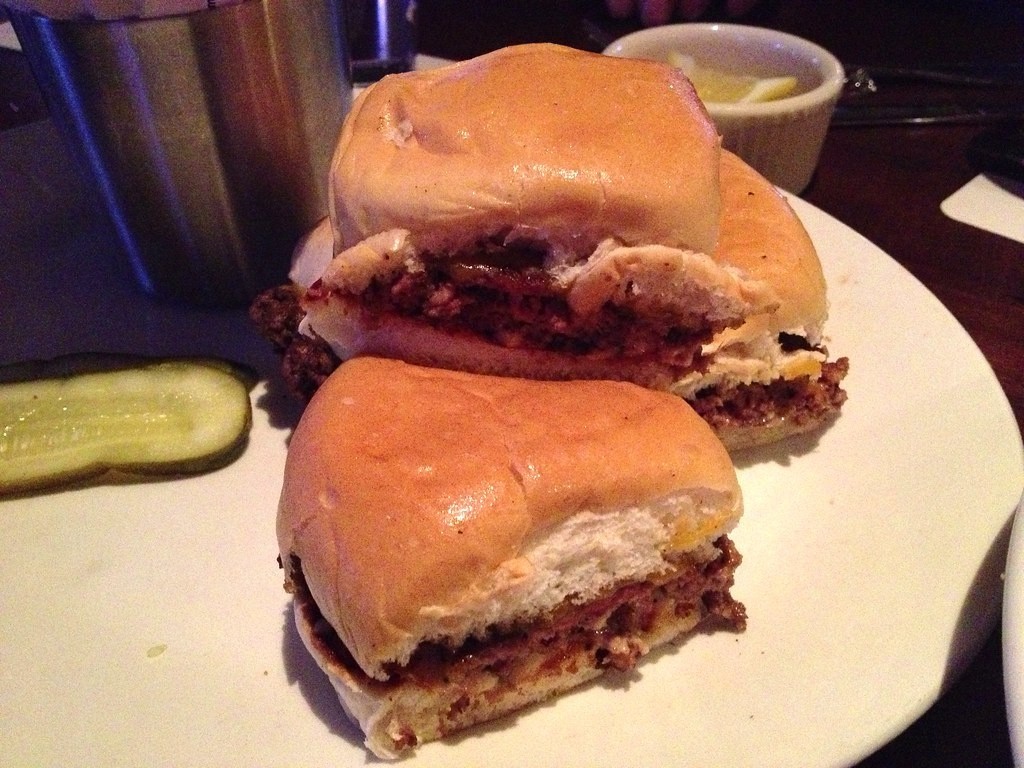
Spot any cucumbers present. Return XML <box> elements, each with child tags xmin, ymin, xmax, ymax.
<box><xmin>0</xmin><ymin>360</ymin><xmax>253</xmax><ymax>492</ymax></box>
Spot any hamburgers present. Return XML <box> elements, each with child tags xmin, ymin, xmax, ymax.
<box><xmin>245</xmin><ymin>42</ymin><xmax>848</xmax><ymax>450</ymax></box>
<box><xmin>276</xmin><ymin>353</ymin><xmax>750</xmax><ymax>761</ymax></box>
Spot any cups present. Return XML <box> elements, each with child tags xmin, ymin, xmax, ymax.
<box><xmin>0</xmin><ymin>0</ymin><xmax>354</xmax><ymax>316</ymax></box>
<box><xmin>336</xmin><ymin>0</ymin><xmax>424</xmax><ymax>84</ymax></box>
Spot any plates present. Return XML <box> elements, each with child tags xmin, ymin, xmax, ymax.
<box><xmin>0</xmin><ymin>85</ymin><xmax>1024</xmax><ymax>768</ymax></box>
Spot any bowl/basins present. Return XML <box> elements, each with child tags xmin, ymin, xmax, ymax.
<box><xmin>601</xmin><ymin>24</ymin><xmax>844</xmax><ymax>195</ymax></box>
